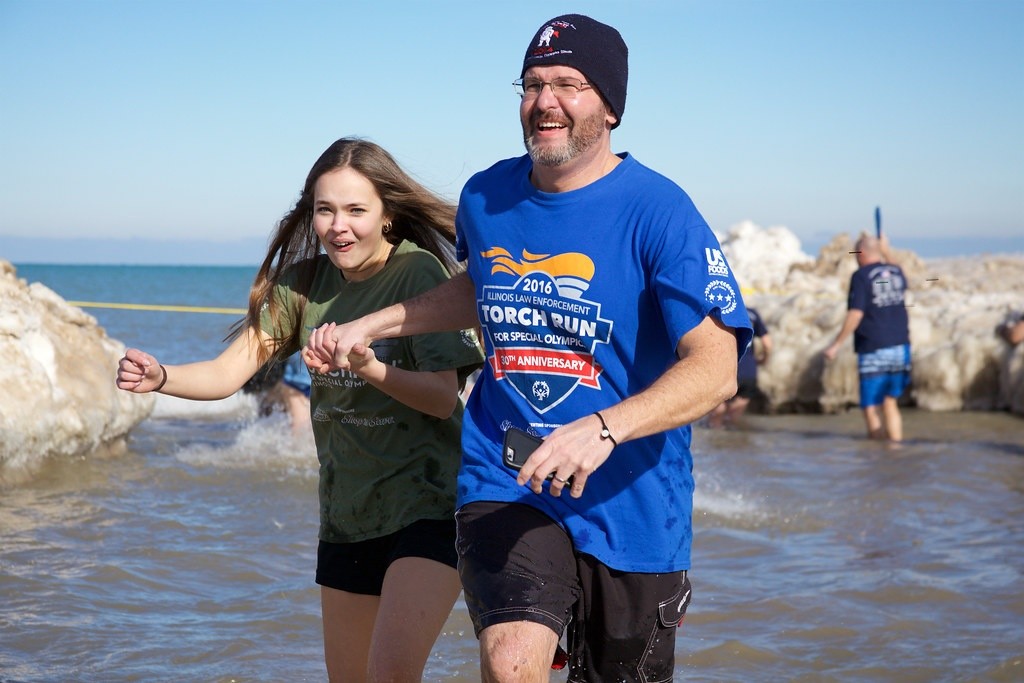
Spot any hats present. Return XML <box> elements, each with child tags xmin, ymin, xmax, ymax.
<box><xmin>520</xmin><ymin>13</ymin><xmax>628</xmax><ymax>131</ymax></box>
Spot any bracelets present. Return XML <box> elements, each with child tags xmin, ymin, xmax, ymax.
<box><xmin>151</xmin><ymin>365</ymin><xmax>168</xmax><ymax>392</ymax></box>
<box><xmin>594</xmin><ymin>412</ymin><xmax>618</xmax><ymax>448</ymax></box>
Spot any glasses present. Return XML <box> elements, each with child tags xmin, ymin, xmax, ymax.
<box><xmin>512</xmin><ymin>77</ymin><xmax>597</xmax><ymax>101</ymax></box>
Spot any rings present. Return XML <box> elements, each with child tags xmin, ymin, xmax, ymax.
<box><xmin>555</xmin><ymin>476</ymin><xmax>567</xmax><ymax>483</ymax></box>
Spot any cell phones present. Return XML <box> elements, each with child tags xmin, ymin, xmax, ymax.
<box><xmin>504</xmin><ymin>427</ymin><xmax>574</xmax><ymax>487</ymax></box>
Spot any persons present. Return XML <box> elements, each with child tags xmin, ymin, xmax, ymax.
<box><xmin>707</xmin><ymin>305</ymin><xmax>771</xmax><ymax>430</ymax></box>
<box><xmin>116</xmin><ymin>137</ymin><xmax>487</xmax><ymax>682</ymax></box>
<box><xmin>825</xmin><ymin>231</ymin><xmax>913</xmax><ymax>441</ymax></box>
<box><xmin>302</xmin><ymin>13</ymin><xmax>754</xmax><ymax>682</ymax></box>
